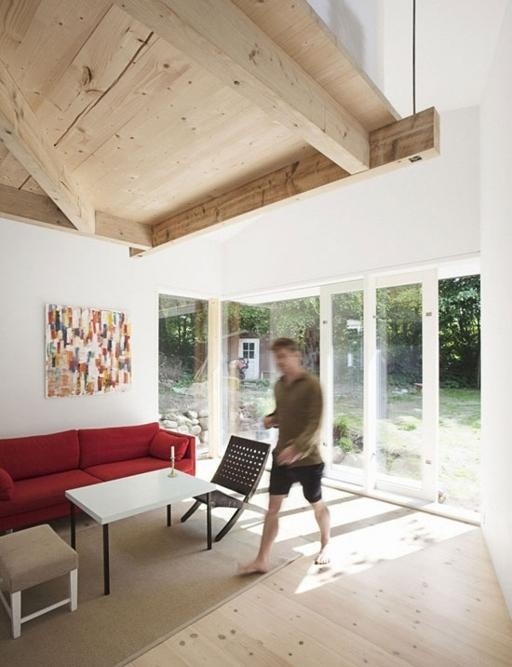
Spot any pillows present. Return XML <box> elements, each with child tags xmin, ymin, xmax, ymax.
<box><xmin>147</xmin><ymin>428</ymin><xmax>189</xmax><ymax>461</ymax></box>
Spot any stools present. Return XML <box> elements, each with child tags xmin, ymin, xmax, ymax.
<box><xmin>0</xmin><ymin>522</ymin><xmax>79</xmax><ymax>639</ymax></box>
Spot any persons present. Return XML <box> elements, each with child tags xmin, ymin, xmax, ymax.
<box><xmin>234</xmin><ymin>336</ymin><xmax>331</xmax><ymax>574</ymax></box>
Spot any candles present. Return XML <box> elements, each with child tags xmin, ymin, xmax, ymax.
<box><xmin>170</xmin><ymin>444</ymin><xmax>174</xmax><ymax>458</ymax></box>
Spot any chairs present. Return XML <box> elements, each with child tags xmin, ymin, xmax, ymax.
<box><xmin>180</xmin><ymin>434</ymin><xmax>271</xmax><ymax>542</ymax></box>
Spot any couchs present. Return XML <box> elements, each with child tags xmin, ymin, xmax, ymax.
<box><xmin>0</xmin><ymin>421</ymin><xmax>195</xmax><ymax>533</ymax></box>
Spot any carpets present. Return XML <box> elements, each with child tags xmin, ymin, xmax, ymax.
<box><xmin>0</xmin><ymin>499</ymin><xmax>303</xmax><ymax>666</ymax></box>
